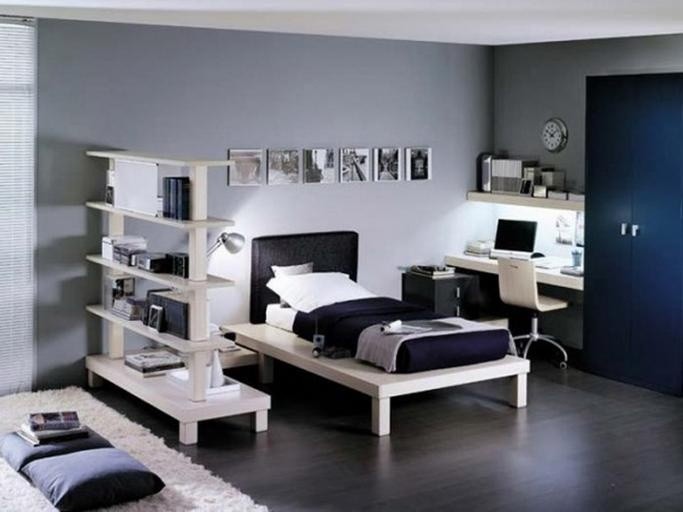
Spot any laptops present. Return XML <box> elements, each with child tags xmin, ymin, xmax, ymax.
<box><xmin>488</xmin><ymin>219</ymin><xmax>539</xmax><ymax>260</ymax></box>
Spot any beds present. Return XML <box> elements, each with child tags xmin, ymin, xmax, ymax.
<box><xmin>221</xmin><ymin>231</ymin><xmax>531</xmax><ymax>438</ymax></box>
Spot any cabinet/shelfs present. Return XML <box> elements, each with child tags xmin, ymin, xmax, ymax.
<box><xmin>585</xmin><ymin>72</ymin><xmax>683</xmax><ymax>396</ymax></box>
<box><xmin>85</xmin><ymin>150</ymin><xmax>271</xmax><ymax>446</ymax></box>
<box><xmin>444</xmin><ymin>191</ymin><xmax>584</xmax><ymax>294</ymax></box>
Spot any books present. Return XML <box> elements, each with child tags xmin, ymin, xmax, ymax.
<box><xmin>162</xmin><ymin>177</ymin><xmax>188</xmax><ymax>221</ymax></box>
<box><xmin>411</xmin><ymin>264</ymin><xmax>456</xmax><ymax>281</ymax></box>
<box><xmin>14</xmin><ymin>411</ymin><xmax>90</xmax><ymax>447</ymax></box>
<box><xmin>464</xmin><ymin>240</ymin><xmax>494</xmax><ymax>257</ymax></box>
<box><xmin>124</xmin><ymin>351</ymin><xmax>187</xmax><ymax>379</ymax></box>
<box><xmin>380</xmin><ymin>319</ymin><xmax>432</xmax><ymax>335</ymax></box>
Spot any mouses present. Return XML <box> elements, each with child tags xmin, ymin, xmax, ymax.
<box><xmin>531</xmin><ymin>252</ymin><xmax>545</xmax><ymax>258</ymax></box>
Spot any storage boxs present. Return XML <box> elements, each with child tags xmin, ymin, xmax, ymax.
<box><xmin>402</xmin><ymin>274</ymin><xmax>481</xmax><ymax>321</ymax></box>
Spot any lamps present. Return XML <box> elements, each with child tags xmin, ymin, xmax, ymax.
<box><xmin>208</xmin><ymin>232</ymin><xmax>245</xmax><ymax>255</ymax></box>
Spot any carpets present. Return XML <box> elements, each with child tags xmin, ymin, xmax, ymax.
<box><xmin>0</xmin><ymin>386</ymin><xmax>269</xmax><ymax>512</ymax></box>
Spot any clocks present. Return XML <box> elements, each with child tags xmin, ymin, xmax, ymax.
<box><xmin>541</xmin><ymin>118</ymin><xmax>568</xmax><ymax>154</ymax></box>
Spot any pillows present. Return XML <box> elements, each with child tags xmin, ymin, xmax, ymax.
<box><xmin>20</xmin><ymin>447</ymin><xmax>166</xmax><ymax>512</ymax></box>
<box><xmin>0</xmin><ymin>411</ymin><xmax>115</xmax><ymax>472</ymax></box>
<box><xmin>265</xmin><ymin>272</ymin><xmax>378</xmax><ymax>314</ymax></box>
<box><xmin>270</xmin><ymin>261</ymin><xmax>315</xmax><ymax>308</ymax></box>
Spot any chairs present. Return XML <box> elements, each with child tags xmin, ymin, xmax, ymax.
<box><xmin>498</xmin><ymin>257</ymin><xmax>569</xmax><ymax>374</ymax></box>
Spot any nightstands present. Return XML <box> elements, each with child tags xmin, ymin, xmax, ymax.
<box><xmin>218</xmin><ymin>336</ymin><xmax>259</xmax><ymax>369</ymax></box>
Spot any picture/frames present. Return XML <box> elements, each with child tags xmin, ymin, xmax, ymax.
<box><xmin>227</xmin><ymin>146</ymin><xmax>432</xmax><ymax>187</ymax></box>
